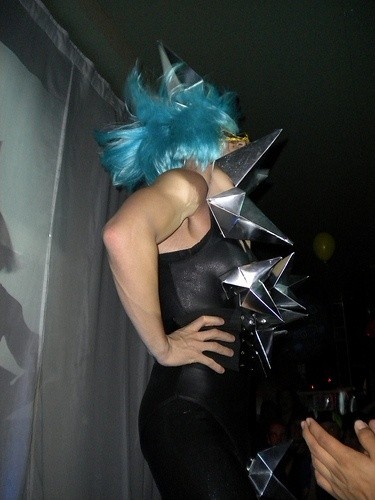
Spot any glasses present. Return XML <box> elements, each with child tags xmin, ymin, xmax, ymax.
<box><xmin>223</xmin><ymin>130</ymin><xmax>249</xmax><ymax>143</ymax></box>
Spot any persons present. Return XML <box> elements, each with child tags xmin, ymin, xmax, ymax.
<box><xmin>101</xmin><ymin>44</ymin><xmax>316</xmax><ymax>500</ymax></box>
